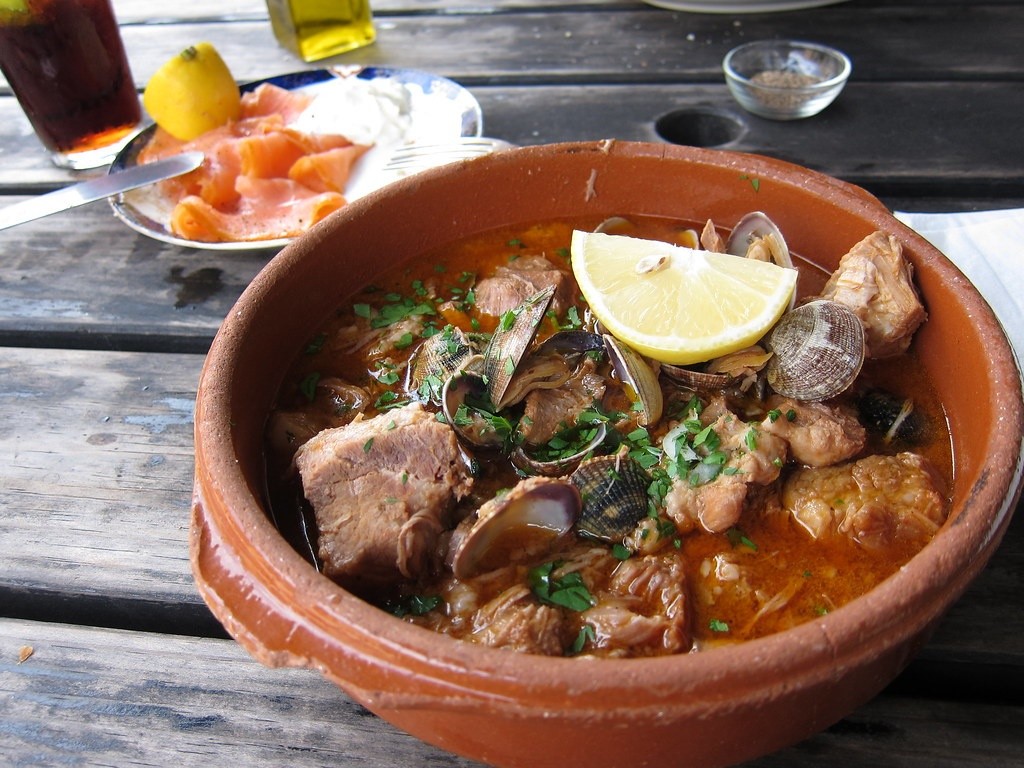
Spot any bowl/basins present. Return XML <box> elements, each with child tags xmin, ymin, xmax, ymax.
<box><xmin>722</xmin><ymin>40</ymin><xmax>852</xmax><ymax>119</ymax></box>
<box><xmin>198</xmin><ymin>142</ymin><xmax>1024</xmax><ymax>768</ymax></box>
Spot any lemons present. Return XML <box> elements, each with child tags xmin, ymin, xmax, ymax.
<box><xmin>143</xmin><ymin>42</ymin><xmax>241</xmax><ymax>141</ymax></box>
<box><xmin>570</xmin><ymin>229</ymin><xmax>798</xmax><ymax>366</ymax></box>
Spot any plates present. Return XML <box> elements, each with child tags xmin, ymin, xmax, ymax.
<box><xmin>108</xmin><ymin>66</ymin><xmax>484</xmax><ymax>254</ymax></box>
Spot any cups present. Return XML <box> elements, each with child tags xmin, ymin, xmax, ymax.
<box><xmin>265</xmin><ymin>0</ymin><xmax>376</xmax><ymax>63</ymax></box>
<box><xmin>0</xmin><ymin>0</ymin><xmax>142</xmax><ymax>168</ymax></box>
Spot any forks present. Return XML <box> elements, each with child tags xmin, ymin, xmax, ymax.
<box><xmin>382</xmin><ymin>136</ymin><xmax>521</xmax><ymax>171</ymax></box>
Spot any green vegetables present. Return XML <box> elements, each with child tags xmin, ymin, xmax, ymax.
<box><xmin>269</xmin><ymin>236</ymin><xmax>797</xmax><ymax>661</ymax></box>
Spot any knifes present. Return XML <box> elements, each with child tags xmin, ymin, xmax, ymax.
<box><xmin>0</xmin><ymin>151</ymin><xmax>204</xmax><ymax>230</ymax></box>
